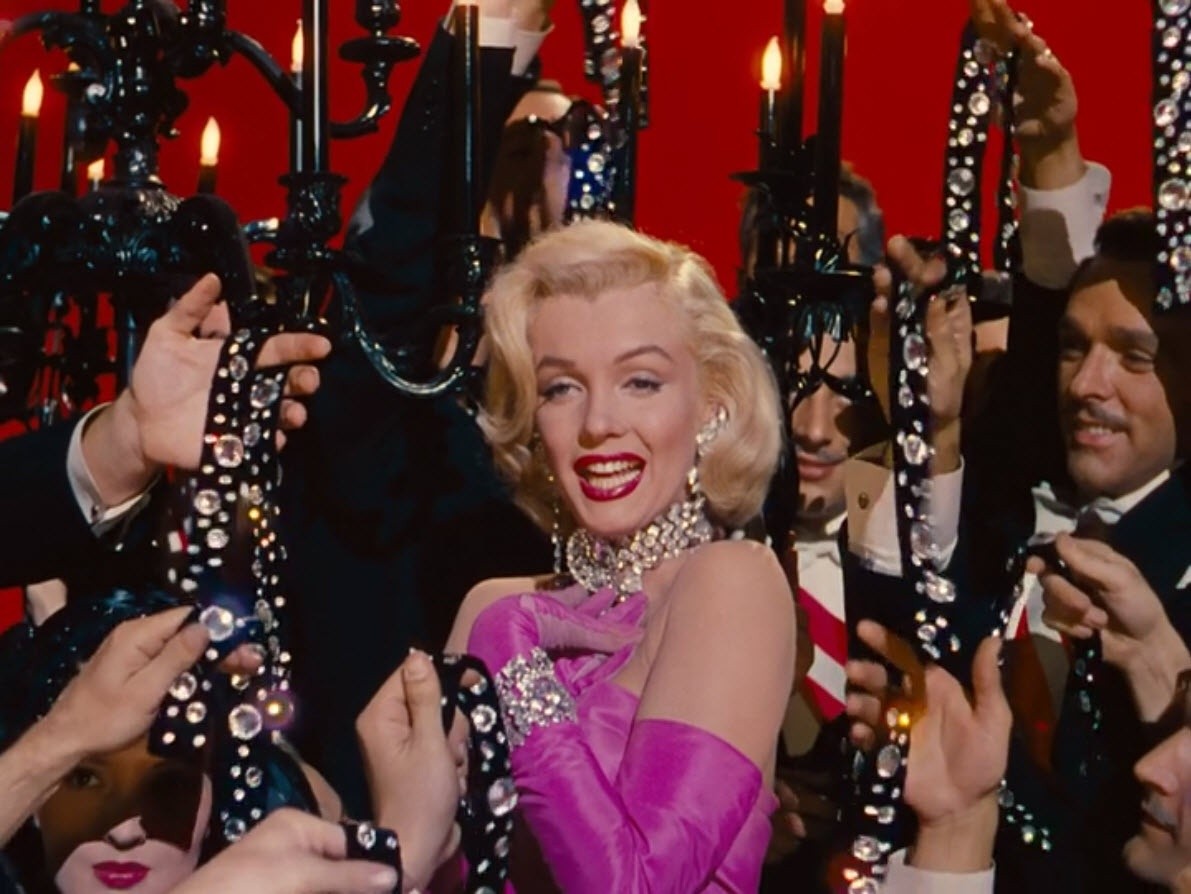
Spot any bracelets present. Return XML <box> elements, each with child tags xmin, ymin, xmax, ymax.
<box><xmin>493</xmin><ymin>645</ymin><xmax>578</xmax><ymax>754</ymax></box>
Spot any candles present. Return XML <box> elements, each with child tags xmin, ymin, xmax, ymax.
<box><xmin>87</xmin><ymin>159</ymin><xmax>104</xmax><ymax>192</ymax></box>
<box><xmin>758</xmin><ymin>38</ymin><xmax>782</xmax><ymax>180</ymax></box>
<box><xmin>289</xmin><ymin>22</ymin><xmax>304</xmax><ymax>173</ymax></box>
<box><xmin>11</xmin><ymin>69</ymin><xmax>44</xmax><ymax>209</ymax></box>
<box><xmin>621</xmin><ymin>0</ymin><xmax>644</xmax><ymax>131</ymax></box>
<box><xmin>196</xmin><ymin>116</ymin><xmax>218</xmax><ymax>195</ymax></box>
<box><xmin>818</xmin><ymin>0</ymin><xmax>845</xmax><ymax>169</ymax></box>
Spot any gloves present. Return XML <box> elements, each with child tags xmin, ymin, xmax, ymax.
<box><xmin>466</xmin><ymin>584</ymin><xmax>647</xmax><ymax>678</ymax></box>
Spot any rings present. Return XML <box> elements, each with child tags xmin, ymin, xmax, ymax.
<box><xmin>1044</xmin><ymin>47</ymin><xmax>1051</xmax><ymax>57</ymax></box>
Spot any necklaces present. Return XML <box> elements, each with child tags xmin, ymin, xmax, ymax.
<box><xmin>565</xmin><ymin>494</ymin><xmax>714</xmax><ymax>603</ymax></box>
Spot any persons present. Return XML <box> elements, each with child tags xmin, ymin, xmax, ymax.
<box><xmin>0</xmin><ymin>0</ymin><xmax>1191</xmax><ymax>894</ymax></box>
